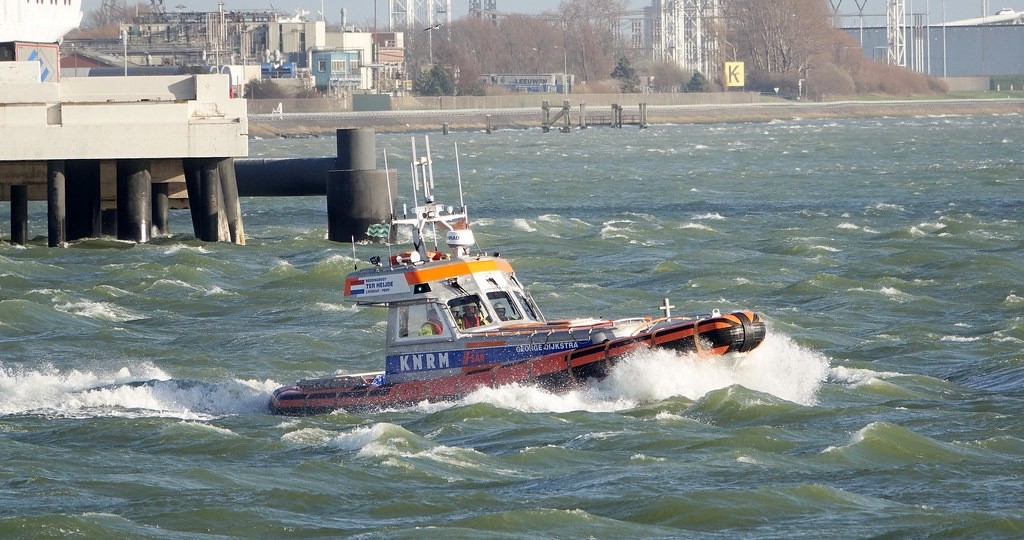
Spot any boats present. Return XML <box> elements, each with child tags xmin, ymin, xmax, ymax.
<box><xmin>267</xmin><ymin>135</ymin><xmax>770</xmax><ymax>417</ymax></box>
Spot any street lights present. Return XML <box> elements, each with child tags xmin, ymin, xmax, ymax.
<box><xmin>553</xmin><ymin>45</ymin><xmax>567</xmax><ymax>78</ymax></box>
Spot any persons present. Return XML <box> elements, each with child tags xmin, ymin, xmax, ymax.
<box><xmin>418</xmin><ymin>306</ymin><xmax>465</xmax><ymax>336</ymax></box>
<box><xmin>481</xmin><ymin>302</ymin><xmax>508</xmax><ymax>325</ymax></box>
<box><xmin>462</xmin><ymin>302</ymin><xmax>483</xmax><ymax>330</ymax></box>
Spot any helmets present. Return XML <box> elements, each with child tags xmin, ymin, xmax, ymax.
<box><xmin>493</xmin><ymin>302</ymin><xmax>506</xmax><ymax>310</ymax></box>
<box><xmin>462</xmin><ymin>302</ymin><xmax>478</xmax><ymax>312</ymax></box>
<box><xmin>450</xmin><ymin>306</ymin><xmax>460</xmax><ymax>312</ymax></box>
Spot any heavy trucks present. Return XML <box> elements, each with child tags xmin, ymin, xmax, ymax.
<box><xmin>474</xmin><ymin>73</ymin><xmax>569</xmax><ymax>94</ymax></box>
